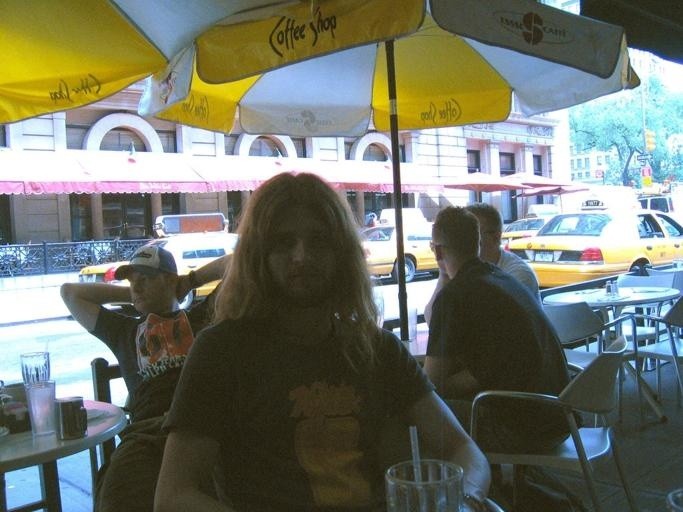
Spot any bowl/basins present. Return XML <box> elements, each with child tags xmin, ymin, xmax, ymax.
<box><xmin>1</xmin><ymin>382</ymin><xmax>26</xmax><ymax>400</ymax></box>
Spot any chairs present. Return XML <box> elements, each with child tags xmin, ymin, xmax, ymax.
<box><xmin>647</xmin><ymin>269</ymin><xmax>683</xmax><ymax>364</ymax></box>
<box><xmin>541</xmin><ymin>301</ymin><xmax>646</xmax><ymax>430</ymax></box>
<box><xmin>91</xmin><ymin>358</ymin><xmax>129</xmax><ymax>466</ymax></box>
<box><xmin>617</xmin><ymin>296</ymin><xmax>683</xmax><ymax>424</ymax></box>
<box><xmin>601</xmin><ymin>273</ymin><xmax>676</xmax><ymax>403</ymax></box>
<box><xmin>469</xmin><ymin>337</ymin><xmax>635</xmax><ymax>512</ymax></box>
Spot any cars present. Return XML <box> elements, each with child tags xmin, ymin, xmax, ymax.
<box><xmin>361</xmin><ymin>224</ymin><xmax>439</xmax><ymax>282</ymax></box>
<box><xmin>505</xmin><ymin>211</ymin><xmax>683</xmax><ymax>288</ymax></box>
<box><xmin>502</xmin><ymin>217</ymin><xmax>549</xmax><ymax>248</ymax></box>
<box><xmin>79</xmin><ymin>233</ymin><xmax>238</xmax><ymax>302</ymax></box>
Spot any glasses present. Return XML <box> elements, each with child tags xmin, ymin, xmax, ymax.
<box><xmin>429</xmin><ymin>241</ymin><xmax>445</xmax><ymax>252</ymax></box>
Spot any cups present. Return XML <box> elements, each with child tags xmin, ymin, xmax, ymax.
<box><xmin>384</xmin><ymin>459</ymin><xmax>464</xmax><ymax>512</ymax></box>
<box><xmin>23</xmin><ymin>380</ymin><xmax>56</xmax><ymax>437</ymax></box>
<box><xmin>408</xmin><ymin>309</ymin><xmax>417</xmax><ymax>340</ymax></box>
<box><xmin>56</xmin><ymin>396</ymin><xmax>88</xmax><ymax>440</ymax></box>
<box><xmin>20</xmin><ymin>351</ymin><xmax>50</xmax><ymax>385</ymax></box>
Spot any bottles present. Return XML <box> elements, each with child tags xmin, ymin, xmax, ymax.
<box><xmin>672</xmin><ymin>259</ymin><xmax>678</xmax><ymax>270</ymax></box>
<box><xmin>606</xmin><ymin>281</ymin><xmax>612</xmax><ymax>294</ymax></box>
<box><xmin>612</xmin><ymin>281</ymin><xmax>618</xmax><ymax>295</ymax></box>
<box><xmin>677</xmin><ymin>258</ymin><xmax>683</xmax><ymax>270</ymax></box>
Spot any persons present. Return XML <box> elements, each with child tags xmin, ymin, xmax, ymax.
<box><xmin>153</xmin><ymin>172</ymin><xmax>491</xmax><ymax>512</ymax></box>
<box><xmin>422</xmin><ymin>206</ymin><xmax>578</xmax><ymax>460</ymax></box>
<box><xmin>60</xmin><ymin>244</ymin><xmax>234</xmax><ymax>511</ymax></box>
<box><xmin>464</xmin><ymin>202</ymin><xmax>540</xmax><ymax>307</ymax></box>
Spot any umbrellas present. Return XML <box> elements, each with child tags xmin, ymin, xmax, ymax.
<box><xmin>0</xmin><ymin>0</ymin><xmax>642</xmax><ymax>337</ymax></box>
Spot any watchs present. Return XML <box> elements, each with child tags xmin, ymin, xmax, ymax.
<box><xmin>189</xmin><ymin>270</ymin><xmax>203</xmax><ymax>288</ymax></box>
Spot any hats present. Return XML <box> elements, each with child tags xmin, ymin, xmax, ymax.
<box><xmin>114</xmin><ymin>244</ymin><xmax>177</xmax><ymax>280</ymax></box>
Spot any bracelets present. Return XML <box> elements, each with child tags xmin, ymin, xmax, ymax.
<box><xmin>461</xmin><ymin>493</ymin><xmax>488</xmax><ymax>512</ymax></box>
